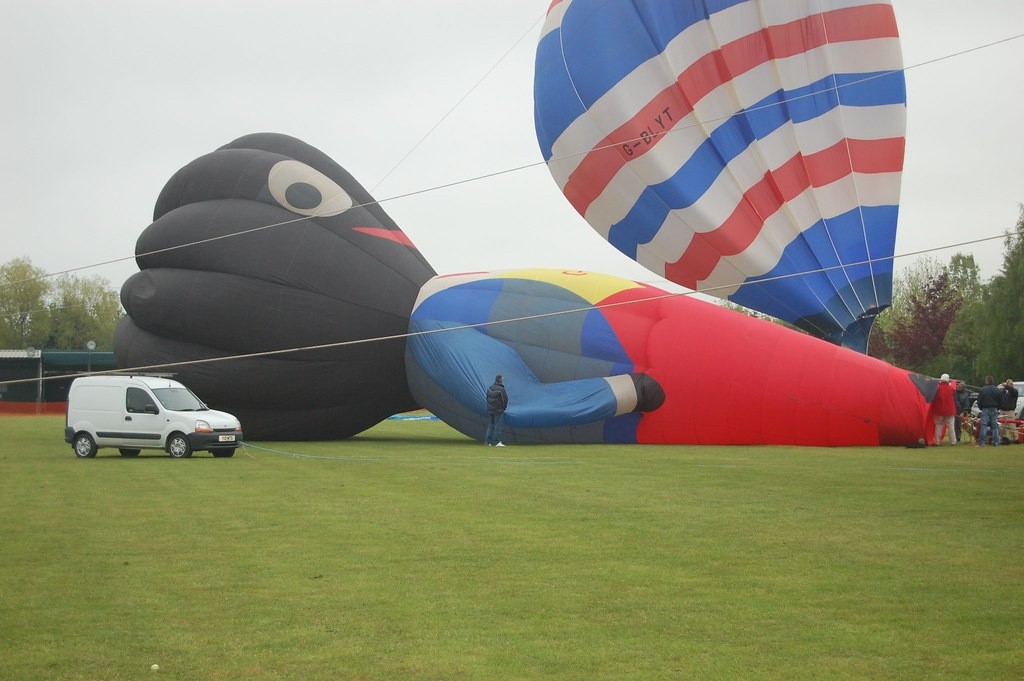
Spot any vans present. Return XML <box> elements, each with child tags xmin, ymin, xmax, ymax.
<box><xmin>63</xmin><ymin>372</ymin><xmax>245</xmax><ymax>460</ymax></box>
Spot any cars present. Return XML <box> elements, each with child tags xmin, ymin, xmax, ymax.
<box><xmin>970</xmin><ymin>381</ymin><xmax>1024</xmax><ymax>421</ymax></box>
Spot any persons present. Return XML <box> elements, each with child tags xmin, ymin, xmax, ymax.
<box><xmin>485</xmin><ymin>375</ymin><xmax>508</xmax><ymax>446</ymax></box>
<box><xmin>932</xmin><ymin>374</ymin><xmax>1019</xmax><ymax>447</ymax></box>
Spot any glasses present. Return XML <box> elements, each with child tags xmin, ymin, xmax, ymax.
<box><xmin>502</xmin><ymin>379</ymin><xmax>503</xmax><ymax>381</ymax></box>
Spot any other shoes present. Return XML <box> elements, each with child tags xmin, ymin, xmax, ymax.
<box><xmin>495</xmin><ymin>441</ymin><xmax>506</xmax><ymax>446</ymax></box>
<box><xmin>1001</xmin><ymin>441</ymin><xmax>1010</xmax><ymax>445</ymax></box>
<box><xmin>975</xmin><ymin>445</ymin><xmax>985</xmax><ymax>448</ymax></box>
<box><xmin>485</xmin><ymin>442</ymin><xmax>492</xmax><ymax>446</ymax></box>
<box><xmin>1012</xmin><ymin>439</ymin><xmax>1019</xmax><ymax>444</ymax></box>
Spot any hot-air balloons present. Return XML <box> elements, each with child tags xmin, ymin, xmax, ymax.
<box><xmin>113</xmin><ymin>131</ymin><xmax>1024</xmax><ymax>448</ymax></box>
<box><xmin>533</xmin><ymin>0</ymin><xmax>908</xmax><ymax>357</ymax></box>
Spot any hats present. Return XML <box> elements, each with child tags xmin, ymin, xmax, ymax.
<box><xmin>936</xmin><ymin>374</ymin><xmax>952</xmax><ymax>383</ymax></box>
